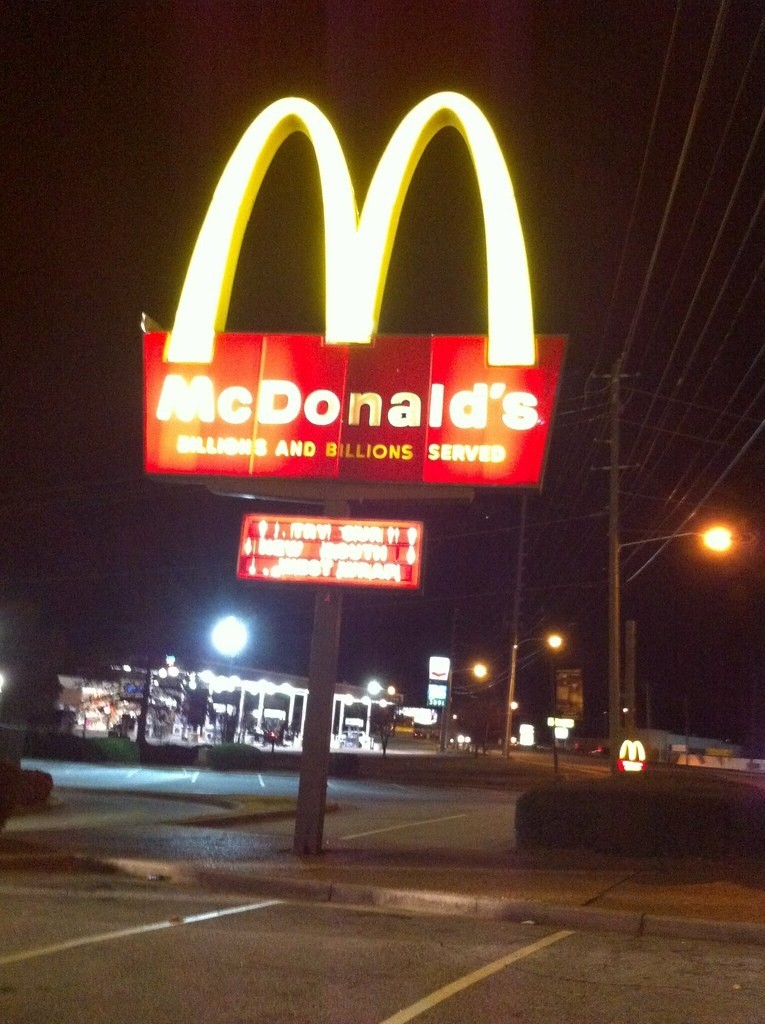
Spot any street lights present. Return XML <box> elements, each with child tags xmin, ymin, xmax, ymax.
<box><xmin>503</xmin><ymin>633</ymin><xmax>563</xmax><ymax>758</ymax></box>
<box><xmin>212</xmin><ymin>616</ymin><xmax>247</xmax><ymax>744</ymax></box>
<box><xmin>440</xmin><ymin>664</ymin><xmax>488</xmax><ymax>750</ymax></box>
<box><xmin>608</xmin><ymin>526</ymin><xmax>733</xmax><ymax>775</ymax></box>
<box><xmin>366</xmin><ymin>681</ymin><xmax>380</xmax><ymax>748</ymax></box>
